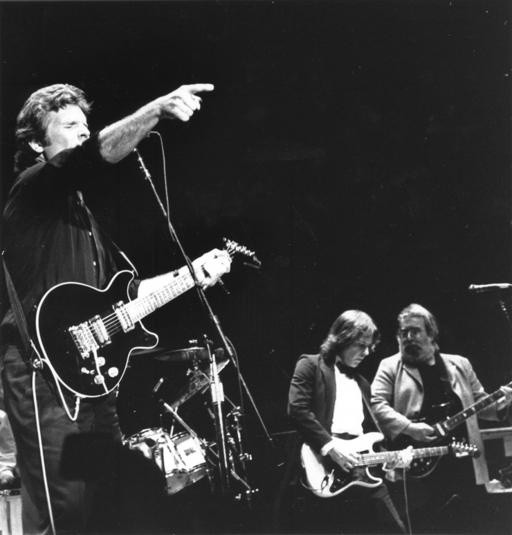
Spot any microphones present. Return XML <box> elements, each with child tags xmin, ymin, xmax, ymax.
<box><xmin>468</xmin><ymin>282</ymin><xmax>512</xmax><ymax>295</ymax></box>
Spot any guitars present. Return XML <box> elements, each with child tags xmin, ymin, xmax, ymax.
<box><xmin>392</xmin><ymin>379</ymin><xmax>512</xmax><ymax>478</ymax></box>
<box><xmin>301</xmin><ymin>431</ymin><xmax>480</xmax><ymax>498</ymax></box>
<box><xmin>33</xmin><ymin>236</ymin><xmax>261</xmax><ymax>400</ymax></box>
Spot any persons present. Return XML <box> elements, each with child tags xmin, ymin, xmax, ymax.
<box><xmin>1</xmin><ymin>81</ymin><xmax>233</xmax><ymax>535</ymax></box>
<box><xmin>370</xmin><ymin>303</ymin><xmax>512</xmax><ymax>535</ymax></box>
<box><xmin>286</xmin><ymin>309</ymin><xmax>382</xmax><ymax>535</ymax></box>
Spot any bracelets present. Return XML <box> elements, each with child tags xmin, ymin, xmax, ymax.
<box><xmin>173</xmin><ymin>268</ymin><xmax>180</xmax><ymax>279</ymax></box>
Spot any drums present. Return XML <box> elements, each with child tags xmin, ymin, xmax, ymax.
<box><xmin>169</xmin><ymin>430</ymin><xmax>211</xmax><ymax>486</ymax></box>
<box><xmin>123</xmin><ymin>427</ymin><xmax>189</xmax><ymax>495</ymax></box>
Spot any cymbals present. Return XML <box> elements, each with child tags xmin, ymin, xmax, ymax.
<box><xmin>155</xmin><ymin>346</ymin><xmax>230</xmax><ymax>363</ymax></box>
<box><xmin>167</xmin><ymin>360</ymin><xmax>232</xmax><ymax>413</ymax></box>
